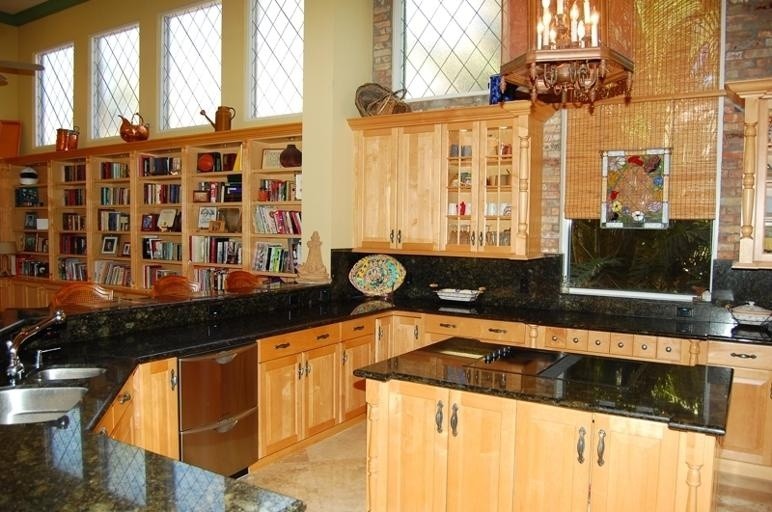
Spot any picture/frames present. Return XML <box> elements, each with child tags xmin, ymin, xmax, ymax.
<box><xmin>599</xmin><ymin>146</ymin><xmax>671</xmax><ymax>231</ymax></box>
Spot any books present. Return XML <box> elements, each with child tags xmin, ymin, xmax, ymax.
<box><xmin>191</xmin><ymin>268</ymin><xmax>229</xmax><ymax>290</ymax></box>
<box><xmin>198</xmin><ymin>174</ymin><xmax>243</xmax><ymax>202</ymax></box>
<box><xmin>142</xmin><ymin>235</ymin><xmax>182</xmax><ymax>261</ymax></box>
<box><xmin>98</xmin><ymin>208</ymin><xmax>130</xmax><ymax>231</ymax></box>
<box><xmin>63</xmin><ymin>189</ymin><xmax>85</xmax><ymax>205</ymax></box>
<box><xmin>98</xmin><ymin>159</ymin><xmax>129</xmax><ymax>179</ymax></box>
<box><xmin>15</xmin><ymin>188</ymin><xmax>39</xmax><ymax>207</ymax></box>
<box><xmin>143</xmin><ymin>182</ymin><xmax>180</xmax><ymax>204</ymax></box>
<box><xmin>142</xmin><ymin>263</ymin><xmax>178</xmax><ymax>290</ymax></box>
<box><xmin>252</xmin><ymin>239</ymin><xmax>299</xmax><ymax>274</ymax></box>
<box><xmin>61</xmin><ymin>234</ymin><xmax>86</xmax><ymax>256</ymax></box>
<box><xmin>16</xmin><ymin>256</ymin><xmax>49</xmax><ymax>277</ymax></box>
<box><xmin>65</xmin><ymin>164</ymin><xmax>86</xmax><ymax>181</ymax></box>
<box><xmin>190</xmin><ymin>235</ymin><xmax>242</xmax><ymax>265</ymax></box>
<box><xmin>252</xmin><ymin>205</ymin><xmax>301</xmax><ymax>234</ymax></box>
<box><xmin>93</xmin><ymin>260</ymin><xmax>130</xmax><ymax>287</ymax></box>
<box><xmin>143</xmin><ymin>153</ymin><xmax>181</xmax><ymax>176</ymax></box>
<box><xmin>258</xmin><ymin>177</ymin><xmax>302</xmax><ymax>201</ymax></box>
<box><xmin>62</xmin><ymin>211</ymin><xmax>86</xmax><ymax>230</ymax></box>
<box><xmin>100</xmin><ymin>187</ymin><xmax>131</xmax><ymax>205</ymax></box>
<box><xmin>58</xmin><ymin>257</ymin><xmax>87</xmax><ymax>281</ymax></box>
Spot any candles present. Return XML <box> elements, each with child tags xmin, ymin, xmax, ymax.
<box><xmin>502</xmin><ymin>0</ymin><xmax>635</xmax><ymax>110</ymax></box>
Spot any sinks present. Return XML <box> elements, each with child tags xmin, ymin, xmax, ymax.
<box><xmin>37</xmin><ymin>368</ymin><xmax>106</xmax><ymax>384</ymax></box>
<box><xmin>0</xmin><ymin>386</ymin><xmax>88</xmax><ymax>426</ymax></box>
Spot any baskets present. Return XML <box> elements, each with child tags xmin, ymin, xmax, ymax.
<box><xmin>355</xmin><ymin>82</ymin><xmax>412</xmax><ymax>118</ymax></box>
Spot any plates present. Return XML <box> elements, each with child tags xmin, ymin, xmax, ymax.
<box><xmin>437</xmin><ymin>288</ymin><xmax>479</xmax><ymax>303</ymax></box>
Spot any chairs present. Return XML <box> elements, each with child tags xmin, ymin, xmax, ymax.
<box><xmin>52</xmin><ymin>279</ymin><xmax>115</xmax><ymax>307</ymax></box>
<box><xmin>224</xmin><ymin>270</ymin><xmax>263</xmax><ymax>289</ymax></box>
<box><xmin>149</xmin><ymin>275</ymin><xmax>202</xmax><ymax>298</ymax></box>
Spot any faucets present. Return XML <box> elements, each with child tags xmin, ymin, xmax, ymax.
<box><xmin>7</xmin><ymin>308</ymin><xmax>67</xmax><ymax>380</ymax></box>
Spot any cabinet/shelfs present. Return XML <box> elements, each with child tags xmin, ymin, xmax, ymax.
<box><xmin>528</xmin><ymin>324</ymin><xmax>708</xmax><ymax>372</ymax></box>
<box><xmin>258</xmin><ymin>322</ymin><xmax>342</xmax><ymax>461</ymax></box>
<box><xmin>376</xmin><ymin>312</ymin><xmax>424</xmax><ymax>363</ymax></box>
<box><xmin>708</xmin><ymin>341</ymin><xmax>771</xmax><ymax>467</ymax></box>
<box><xmin>343</xmin><ymin>311</ymin><xmax>393</xmax><ymax>430</ymax></box>
<box><xmin>1</xmin><ymin>121</ymin><xmax>304</xmax><ymax>310</ymax></box>
<box><xmin>346</xmin><ymin>109</ymin><xmax>442</xmax><ymax>257</ymax></box>
<box><xmin>180</xmin><ymin>344</ymin><xmax>257</xmax><ymax>480</ymax></box>
<box><xmin>516</xmin><ymin>399</ymin><xmax>723</xmax><ymax>512</ymax></box>
<box><xmin>442</xmin><ymin>102</ymin><xmax>544</xmax><ymax>260</ymax></box>
<box><xmin>425</xmin><ymin>313</ymin><xmax>527</xmax><ymax>361</ymax></box>
<box><xmin>365</xmin><ymin>376</ymin><xmax>515</xmax><ymax>512</ymax></box>
<box><xmin>93</xmin><ymin>356</ymin><xmax>179</xmax><ymax>462</ymax></box>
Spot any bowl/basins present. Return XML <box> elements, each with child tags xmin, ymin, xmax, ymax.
<box><xmin>726</xmin><ymin>302</ymin><xmax>772</xmax><ymax>326</ymax></box>
